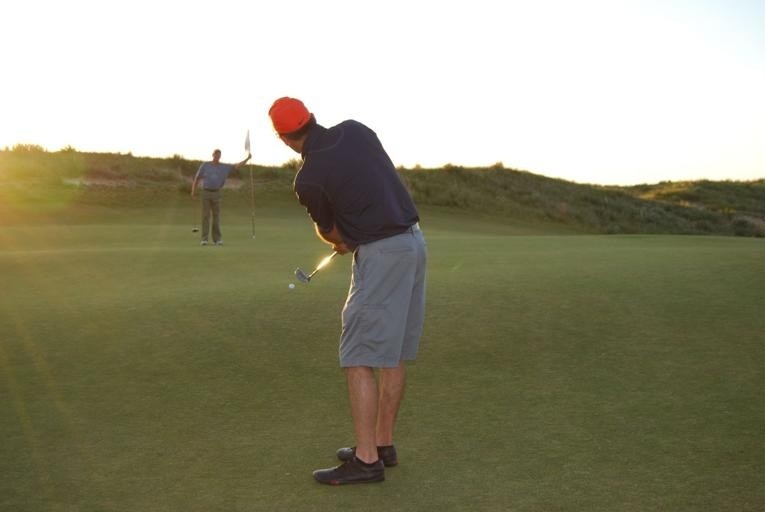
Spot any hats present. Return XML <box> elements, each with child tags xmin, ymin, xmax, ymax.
<box><xmin>268</xmin><ymin>96</ymin><xmax>311</xmax><ymax>134</ymax></box>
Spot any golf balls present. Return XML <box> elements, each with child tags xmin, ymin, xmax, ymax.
<box><xmin>289</xmin><ymin>283</ymin><xmax>297</xmax><ymax>289</ymax></box>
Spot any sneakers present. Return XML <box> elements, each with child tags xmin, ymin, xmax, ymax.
<box><xmin>312</xmin><ymin>444</ymin><xmax>399</xmax><ymax>486</ymax></box>
<box><xmin>200</xmin><ymin>239</ymin><xmax>209</xmax><ymax>246</ymax></box>
<box><xmin>212</xmin><ymin>240</ymin><xmax>223</xmax><ymax>245</ymax></box>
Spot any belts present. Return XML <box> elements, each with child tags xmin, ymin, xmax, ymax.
<box><xmin>203</xmin><ymin>188</ymin><xmax>220</xmax><ymax>191</ymax></box>
<box><xmin>406</xmin><ymin>221</ymin><xmax>421</xmax><ymax>233</ymax></box>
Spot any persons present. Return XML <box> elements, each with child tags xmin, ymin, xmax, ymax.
<box><xmin>268</xmin><ymin>97</ymin><xmax>426</xmax><ymax>487</ymax></box>
<box><xmin>191</xmin><ymin>150</ymin><xmax>252</xmax><ymax>245</ymax></box>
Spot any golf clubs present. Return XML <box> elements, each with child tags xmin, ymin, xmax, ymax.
<box><xmin>295</xmin><ymin>251</ymin><xmax>337</xmax><ymax>283</ymax></box>
<box><xmin>193</xmin><ymin>198</ymin><xmax>200</xmax><ymax>232</ymax></box>
